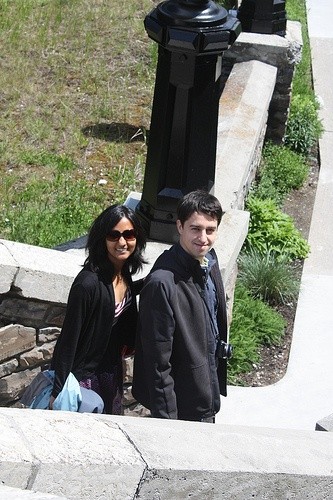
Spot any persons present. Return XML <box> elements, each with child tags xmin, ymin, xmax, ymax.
<box><xmin>49</xmin><ymin>204</ymin><xmax>146</xmax><ymax>416</ymax></box>
<box><xmin>131</xmin><ymin>190</ymin><xmax>232</xmax><ymax>423</ymax></box>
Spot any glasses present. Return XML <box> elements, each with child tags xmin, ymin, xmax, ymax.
<box><xmin>106</xmin><ymin>230</ymin><xmax>137</xmax><ymax>242</ymax></box>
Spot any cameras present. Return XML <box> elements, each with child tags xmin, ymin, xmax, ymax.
<box><xmin>215</xmin><ymin>340</ymin><xmax>236</xmax><ymax>359</ymax></box>
